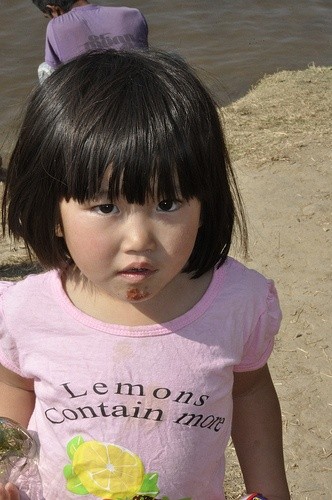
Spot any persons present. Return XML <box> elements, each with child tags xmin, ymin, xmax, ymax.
<box><xmin>0</xmin><ymin>48</ymin><xmax>291</xmax><ymax>500</ymax></box>
<box><xmin>32</xmin><ymin>0</ymin><xmax>149</xmax><ymax>85</ymax></box>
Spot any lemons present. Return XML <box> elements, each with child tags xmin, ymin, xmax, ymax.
<box><xmin>72</xmin><ymin>441</ymin><xmax>144</xmax><ymax>500</ymax></box>
